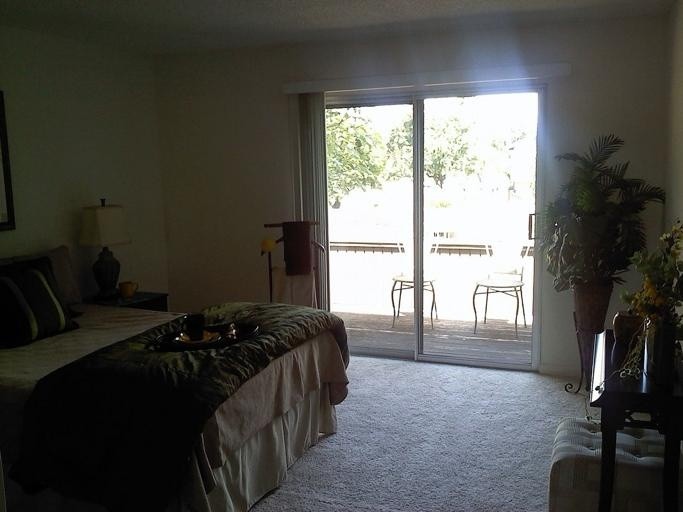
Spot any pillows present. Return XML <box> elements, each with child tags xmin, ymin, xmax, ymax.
<box><xmin>0</xmin><ymin>259</ymin><xmax>67</xmax><ymax>349</ymax></box>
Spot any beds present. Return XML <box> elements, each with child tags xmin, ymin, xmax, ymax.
<box><xmin>0</xmin><ymin>246</ymin><xmax>337</xmax><ymax>512</ymax></box>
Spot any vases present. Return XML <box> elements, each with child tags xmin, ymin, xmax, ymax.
<box><xmin>635</xmin><ymin>325</ymin><xmax>677</xmax><ymax>397</ymax></box>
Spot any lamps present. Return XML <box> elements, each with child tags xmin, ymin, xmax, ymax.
<box><xmin>74</xmin><ymin>199</ymin><xmax>131</xmax><ymax>296</ymax></box>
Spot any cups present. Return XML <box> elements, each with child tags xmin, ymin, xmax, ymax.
<box><xmin>177</xmin><ymin>313</ymin><xmax>205</xmax><ymax>340</ymax></box>
<box><xmin>118</xmin><ymin>280</ymin><xmax>138</xmax><ymax>299</ymax></box>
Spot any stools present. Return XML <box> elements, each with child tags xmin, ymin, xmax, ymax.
<box><xmin>549</xmin><ymin>417</ymin><xmax>665</xmax><ymax>511</ymax></box>
<box><xmin>391</xmin><ymin>265</ymin><xmax>527</xmax><ymax>341</ymax></box>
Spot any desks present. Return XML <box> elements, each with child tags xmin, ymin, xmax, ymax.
<box><xmin>592</xmin><ymin>331</ymin><xmax>682</xmax><ymax>510</ymax></box>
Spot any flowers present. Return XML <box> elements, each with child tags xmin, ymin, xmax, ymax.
<box><xmin>621</xmin><ymin>219</ymin><xmax>679</xmax><ymax>378</ymax></box>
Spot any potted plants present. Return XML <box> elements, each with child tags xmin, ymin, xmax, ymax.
<box><xmin>536</xmin><ymin>133</ymin><xmax>666</xmax><ymax>396</ymax></box>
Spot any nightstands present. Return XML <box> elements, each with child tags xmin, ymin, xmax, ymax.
<box><xmin>90</xmin><ymin>291</ymin><xmax>170</xmax><ymax>313</ymax></box>
